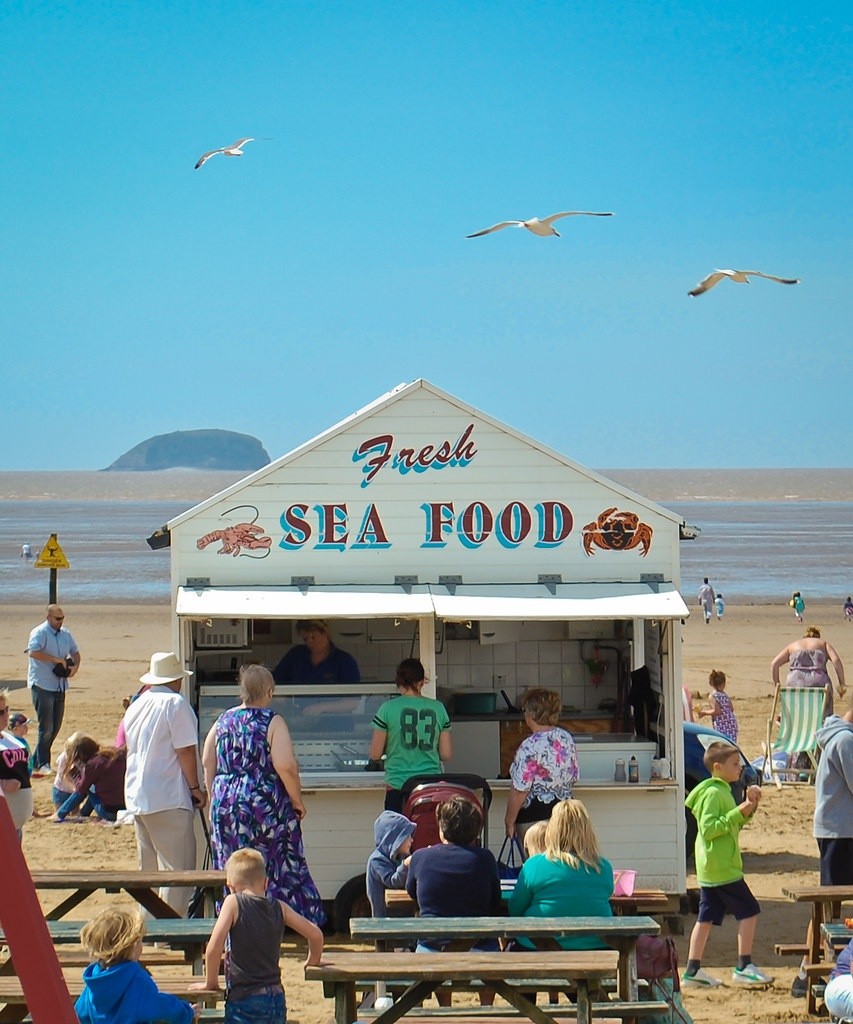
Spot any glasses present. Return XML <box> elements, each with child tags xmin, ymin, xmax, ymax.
<box><xmin>0</xmin><ymin>706</ymin><xmax>9</xmax><ymax>716</ymax></box>
<box><xmin>50</xmin><ymin>615</ymin><xmax>65</xmax><ymax>621</ymax></box>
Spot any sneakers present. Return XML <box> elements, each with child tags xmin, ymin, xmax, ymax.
<box><xmin>681</xmin><ymin>969</ymin><xmax>723</xmax><ymax>988</ymax></box>
<box><xmin>791</xmin><ymin>976</ymin><xmax>828</xmax><ymax>999</ymax></box>
<box><xmin>732</xmin><ymin>963</ymin><xmax>774</xmax><ymax>984</ymax></box>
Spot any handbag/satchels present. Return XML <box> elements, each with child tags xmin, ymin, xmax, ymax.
<box><xmin>497</xmin><ymin>834</ymin><xmax>526</xmax><ymax>880</ymax></box>
<box><xmin>52</xmin><ymin>658</ymin><xmax>74</xmax><ymax>677</ymax></box>
<box><xmin>183</xmin><ymin>845</ymin><xmax>210</xmax><ymax>962</ymax></box>
<box><xmin>635</xmin><ymin>935</ymin><xmax>680</xmax><ymax>993</ymax></box>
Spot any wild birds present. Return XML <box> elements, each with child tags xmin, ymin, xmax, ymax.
<box><xmin>688</xmin><ymin>269</ymin><xmax>801</xmax><ymax>297</ymax></box>
<box><xmin>193</xmin><ymin>137</ymin><xmax>255</xmax><ymax>170</ymax></box>
<box><xmin>465</xmin><ymin>212</ymin><xmax>614</xmax><ymax>239</ymax></box>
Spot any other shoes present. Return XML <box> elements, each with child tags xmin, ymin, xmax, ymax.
<box><xmin>39</xmin><ymin>763</ymin><xmax>56</xmax><ymax>775</ymax></box>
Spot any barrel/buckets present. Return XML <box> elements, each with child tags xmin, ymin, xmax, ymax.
<box><xmin>612</xmin><ymin>869</ymin><xmax>637</xmax><ymax>897</ymax></box>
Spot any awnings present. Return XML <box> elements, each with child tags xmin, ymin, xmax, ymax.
<box><xmin>172</xmin><ymin>583</ymin><xmax>694</xmax><ymax>622</ymax></box>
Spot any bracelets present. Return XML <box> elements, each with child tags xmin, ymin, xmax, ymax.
<box><xmin>188</xmin><ymin>783</ymin><xmax>200</xmax><ymax>791</ymax></box>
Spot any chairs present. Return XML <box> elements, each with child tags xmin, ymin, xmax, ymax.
<box><xmin>758</xmin><ymin>683</ymin><xmax>829</xmax><ymax>791</ymax></box>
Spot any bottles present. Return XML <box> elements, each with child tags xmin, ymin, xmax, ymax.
<box><xmin>614</xmin><ymin>756</ymin><xmax>627</xmax><ymax>781</ymax></box>
<box><xmin>650</xmin><ymin>754</ymin><xmax>660</xmax><ymax>778</ymax></box>
<box><xmin>628</xmin><ymin>755</ymin><xmax>638</xmax><ymax>782</ymax></box>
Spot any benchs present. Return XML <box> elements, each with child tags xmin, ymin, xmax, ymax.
<box><xmin>773</xmin><ymin>883</ymin><xmax>853</xmax><ymax>1001</ymax></box>
<box><xmin>0</xmin><ymin>855</ymin><xmax>696</xmax><ymax>1024</ymax></box>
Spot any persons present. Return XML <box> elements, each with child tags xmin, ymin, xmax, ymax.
<box><xmin>715</xmin><ymin>593</ymin><xmax>725</xmax><ymax>621</ymax></box>
<box><xmin>21</xmin><ymin>542</ymin><xmax>31</xmax><ymax>557</ymax></box>
<box><xmin>698</xmin><ymin>669</ymin><xmax>738</xmax><ymax>744</ymax></box>
<box><xmin>404</xmin><ymin>794</ymin><xmax>504</xmax><ymax>1007</ymax></box>
<box><xmin>371</xmin><ymin>660</ymin><xmax>452</xmax><ymax>818</ymax></box>
<box><xmin>793</xmin><ymin>592</ymin><xmax>806</xmax><ymax>622</ymax></box>
<box><xmin>202</xmin><ymin>665</ymin><xmax>305</xmax><ymax>949</ymax></box>
<box><xmin>699</xmin><ymin>577</ymin><xmax>715</xmax><ymax>623</ymax></box>
<box><xmin>123</xmin><ymin>652</ymin><xmax>207</xmax><ymax>948</ymax></box>
<box><xmin>365</xmin><ymin>810</ymin><xmax>416</xmax><ymax>919</ymax></box>
<box><xmin>843</xmin><ymin>597</ymin><xmax>853</xmax><ymax>622</ymax></box>
<box><xmin>771</xmin><ymin>626</ymin><xmax>846</xmax><ymax>717</ymax></box>
<box><xmin>508</xmin><ymin>800</ymin><xmax>615</xmax><ymax>1006</ymax></box>
<box><xmin>115</xmin><ymin>694</ymin><xmax>131</xmax><ymax>748</ymax></box>
<box><xmin>824</xmin><ymin>931</ymin><xmax>853</xmax><ymax>1023</ymax></box>
<box><xmin>790</xmin><ymin>706</ymin><xmax>853</xmax><ymax>998</ymax></box>
<box><xmin>189</xmin><ymin>847</ymin><xmax>335</xmax><ymax>1024</ymax></box>
<box><xmin>45</xmin><ymin>737</ymin><xmax>126</xmax><ymax>822</ymax></box>
<box><xmin>523</xmin><ymin>819</ymin><xmax>550</xmax><ymax>858</ymax></box>
<box><xmin>505</xmin><ymin>691</ymin><xmax>579</xmax><ymax>862</ymax></box>
<box><xmin>0</xmin><ymin>691</ymin><xmax>33</xmax><ymax>849</ymax></box>
<box><xmin>679</xmin><ymin>742</ymin><xmax>776</xmax><ymax>990</ymax></box>
<box><xmin>270</xmin><ymin>619</ymin><xmax>360</xmax><ymax>730</ymax></box>
<box><xmin>51</xmin><ymin>732</ymin><xmax>89</xmax><ymax>814</ymax></box>
<box><xmin>7</xmin><ymin>713</ymin><xmax>33</xmax><ymax>777</ymax></box>
<box><xmin>27</xmin><ymin>605</ymin><xmax>81</xmax><ymax>775</ymax></box>
<box><xmin>74</xmin><ymin>906</ymin><xmax>198</xmax><ymax>1024</ymax></box>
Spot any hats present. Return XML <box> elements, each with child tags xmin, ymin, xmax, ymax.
<box><xmin>8</xmin><ymin>713</ymin><xmax>31</xmax><ymax>731</ymax></box>
<box><xmin>139</xmin><ymin>652</ymin><xmax>194</xmax><ymax>685</ymax></box>
